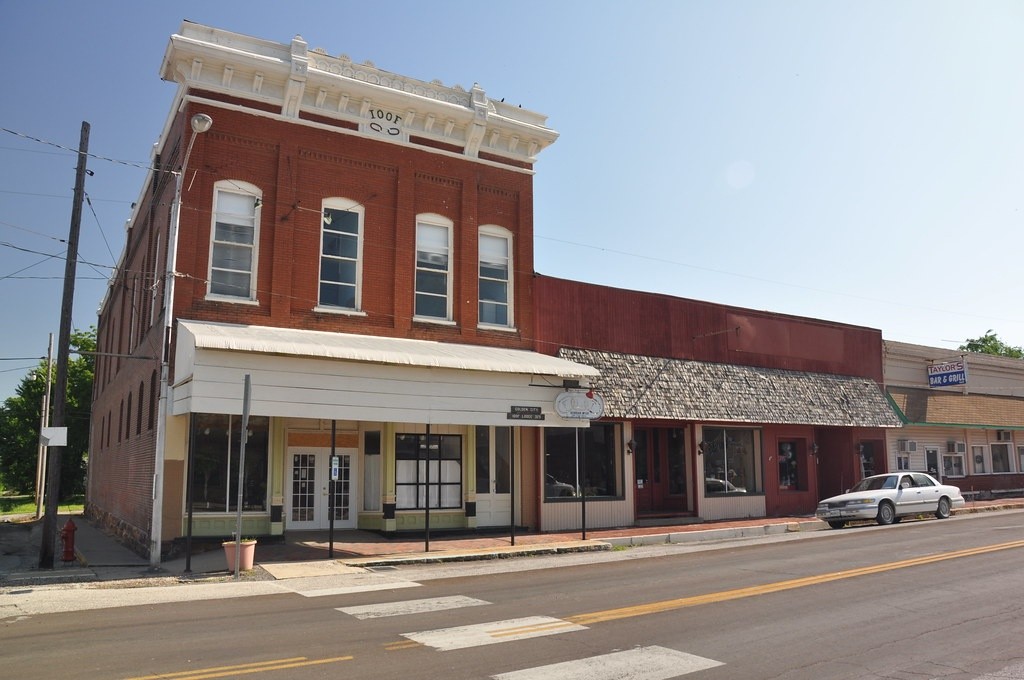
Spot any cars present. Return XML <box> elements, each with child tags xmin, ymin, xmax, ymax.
<box><xmin>706</xmin><ymin>477</ymin><xmax>747</xmax><ymax>495</ymax></box>
<box><xmin>546</xmin><ymin>473</ymin><xmax>576</xmax><ymax>497</ymax></box>
<box><xmin>815</xmin><ymin>471</ymin><xmax>965</xmax><ymax>529</ymax></box>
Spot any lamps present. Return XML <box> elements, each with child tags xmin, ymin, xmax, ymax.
<box><xmin>253</xmin><ymin>198</ymin><xmax>263</xmax><ymax>210</ymax></box>
<box><xmin>627</xmin><ymin>439</ymin><xmax>638</xmax><ymax>454</ymax></box>
<box><xmin>698</xmin><ymin>440</ymin><xmax>709</xmax><ymax>455</ymax></box>
<box><xmin>809</xmin><ymin>442</ymin><xmax>819</xmax><ymax>456</ymax></box>
<box><xmin>855</xmin><ymin>442</ymin><xmax>864</xmax><ymax>455</ymax></box>
<box><xmin>324</xmin><ymin>212</ymin><xmax>333</xmax><ymax>225</ymax></box>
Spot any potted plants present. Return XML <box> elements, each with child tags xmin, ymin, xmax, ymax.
<box><xmin>222</xmin><ymin>534</ymin><xmax>258</xmax><ymax>571</ymax></box>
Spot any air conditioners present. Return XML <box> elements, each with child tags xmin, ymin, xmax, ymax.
<box><xmin>999</xmin><ymin>431</ymin><xmax>1012</xmax><ymax>441</ymax></box>
<box><xmin>948</xmin><ymin>441</ymin><xmax>966</xmax><ymax>454</ymax></box>
<box><xmin>897</xmin><ymin>439</ymin><xmax>918</xmax><ymax>452</ymax></box>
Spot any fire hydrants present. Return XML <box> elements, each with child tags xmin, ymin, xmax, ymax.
<box><xmin>59</xmin><ymin>516</ymin><xmax>78</xmax><ymax>562</ymax></box>
